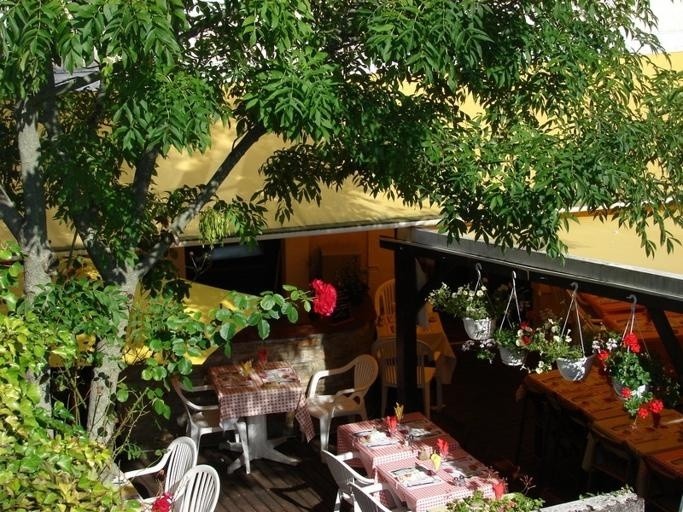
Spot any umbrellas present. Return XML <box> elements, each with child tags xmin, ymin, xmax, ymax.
<box><xmin>1</xmin><ymin>255</ymin><xmax>266</xmax><ymax>366</ymax></box>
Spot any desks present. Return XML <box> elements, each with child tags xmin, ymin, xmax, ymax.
<box><xmin>514</xmin><ymin>356</ymin><xmax>682</xmax><ymax>511</ymax></box>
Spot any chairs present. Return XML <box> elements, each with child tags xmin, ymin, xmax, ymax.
<box><xmin>110</xmin><ymin>348</ymin><xmax>253</xmax><ymax>512</ymax></box>
<box><xmin>298</xmin><ymin>277</ymin><xmax>440</xmax><ymax>512</ymax></box>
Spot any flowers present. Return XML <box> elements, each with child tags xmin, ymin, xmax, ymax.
<box><xmin>424</xmin><ymin>277</ymin><xmax>666</xmax><ymax>421</ymax></box>
